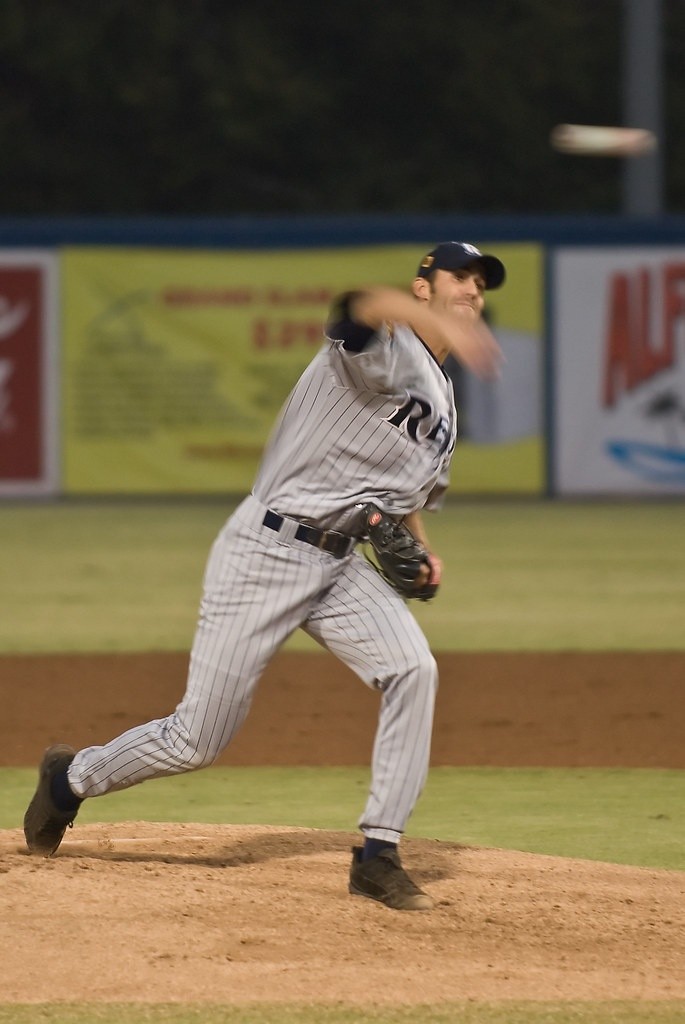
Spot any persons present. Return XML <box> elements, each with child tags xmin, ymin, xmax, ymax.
<box><xmin>23</xmin><ymin>240</ymin><xmax>507</xmax><ymax>910</ymax></box>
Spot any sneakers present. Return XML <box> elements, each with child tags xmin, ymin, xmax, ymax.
<box><xmin>348</xmin><ymin>845</ymin><xmax>435</xmax><ymax>911</ymax></box>
<box><xmin>24</xmin><ymin>743</ymin><xmax>85</xmax><ymax>857</ymax></box>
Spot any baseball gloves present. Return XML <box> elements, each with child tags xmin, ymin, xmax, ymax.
<box><xmin>364</xmin><ymin>501</ymin><xmax>441</xmax><ymax>602</ymax></box>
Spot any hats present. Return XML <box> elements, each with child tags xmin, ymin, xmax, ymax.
<box><xmin>418</xmin><ymin>240</ymin><xmax>506</xmax><ymax>290</ymax></box>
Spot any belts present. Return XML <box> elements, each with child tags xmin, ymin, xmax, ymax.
<box><xmin>262</xmin><ymin>509</ymin><xmax>356</xmax><ymax>559</ymax></box>
